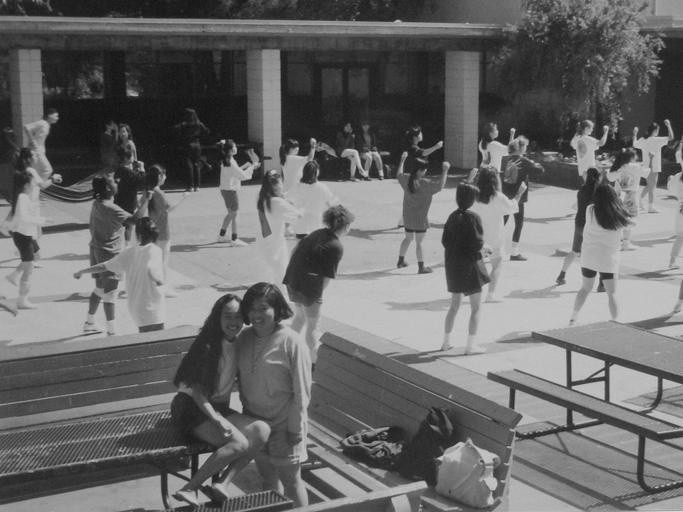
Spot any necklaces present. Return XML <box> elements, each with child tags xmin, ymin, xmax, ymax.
<box><xmin>252</xmin><ymin>326</ymin><xmax>279</xmax><ymax>374</ymax></box>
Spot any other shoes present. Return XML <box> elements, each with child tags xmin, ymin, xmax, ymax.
<box><xmin>399</xmin><ymin>262</ymin><xmax>408</xmax><ymax>268</ymax></box>
<box><xmin>621</xmin><ymin>244</ymin><xmax>640</xmax><ymax>250</ymax></box>
<box><xmin>556</xmin><ymin>279</ymin><xmax>566</xmax><ymax>285</ymax></box>
<box><xmin>669</xmin><ymin>263</ymin><xmax>680</xmax><ymax>269</ymax></box>
<box><xmin>441</xmin><ymin>343</ymin><xmax>455</xmax><ymax>351</ymax></box>
<box><xmin>417</xmin><ymin>268</ymin><xmax>433</xmax><ymax>273</ymax></box>
<box><xmin>485</xmin><ymin>297</ymin><xmax>503</xmax><ymax>303</ymax></box>
<box><xmin>511</xmin><ymin>254</ymin><xmax>526</xmax><ymax>261</ymax></box>
<box><xmin>597</xmin><ymin>284</ymin><xmax>606</xmax><ymax>291</ymax></box>
<box><xmin>674</xmin><ymin>305</ymin><xmax>682</xmax><ymax>313</ymax></box>
<box><xmin>466</xmin><ymin>345</ymin><xmax>486</xmax><ymax>353</ymax></box>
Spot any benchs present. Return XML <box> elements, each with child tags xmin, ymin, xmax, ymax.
<box><xmin>0</xmin><ymin>325</ymin><xmax>237</xmax><ymax>511</ymax></box>
<box><xmin>485</xmin><ymin>367</ymin><xmax>682</xmax><ymax>490</ymax></box>
<box><xmin>300</xmin><ymin>330</ymin><xmax>522</xmax><ymax>512</ymax></box>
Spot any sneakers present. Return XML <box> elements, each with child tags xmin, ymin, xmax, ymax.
<box><xmin>349</xmin><ymin>174</ymin><xmax>384</xmax><ymax>182</ymax></box>
<box><xmin>0</xmin><ymin>220</ymin><xmax>103</xmax><ymax>334</ymax></box>
<box><xmin>216</xmin><ymin>234</ymin><xmax>248</xmax><ymax>247</ymax></box>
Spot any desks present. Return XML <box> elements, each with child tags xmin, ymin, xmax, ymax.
<box><xmin>531</xmin><ymin>320</ymin><xmax>682</xmax><ymax>428</ymax></box>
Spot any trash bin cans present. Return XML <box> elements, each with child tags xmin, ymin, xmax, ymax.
<box><xmin>234</xmin><ymin>143</ymin><xmax>261</xmax><ymax>184</ymax></box>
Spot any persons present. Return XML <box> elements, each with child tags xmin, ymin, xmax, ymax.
<box><xmin>398</xmin><ymin>127</ymin><xmax>443</xmax><ymax>228</ymax></box>
<box><xmin>441</xmin><ymin>181</ymin><xmax>491</xmax><ymax>355</ymax></box>
<box><xmin>74</xmin><ymin>217</ymin><xmax>165</xmax><ymax>332</ymax></box>
<box><xmin>216</xmin><ymin>139</ymin><xmax>255</xmax><ymax>248</ymax></box>
<box><xmin>500</xmin><ymin>135</ymin><xmax>545</xmax><ymax>260</ymax></box>
<box><xmin>397</xmin><ymin>151</ymin><xmax>450</xmax><ymax>272</ymax></box>
<box><xmin>283</xmin><ymin>205</ymin><xmax>355</xmax><ymax>368</ymax></box>
<box><xmin>472</xmin><ymin>166</ymin><xmax>527</xmax><ymax>303</ymax></box>
<box><xmin>6</xmin><ymin>170</ymin><xmax>39</xmax><ymax>310</ymax></box>
<box><xmin>115</xmin><ymin>144</ymin><xmax>146</xmax><ymax>251</ymax></box>
<box><xmin>356</xmin><ymin>120</ymin><xmax>385</xmax><ymax>181</ymax></box>
<box><xmin>633</xmin><ymin>119</ymin><xmax>674</xmax><ymax>213</ymax></box>
<box><xmin>100</xmin><ymin>119</ymin><xmax>137</xmax><ymax>178</ymax></box>
<box><xmin>279</xmin><ymin>138</ymin><xmax>316</xmax><ymax>200</ymax></box>
<box><xmin>23</xmin><ymin>109</ymin><xmax>59</xmax><ymax>182</ymax></box>
<box><xmin>171</xmin><ymin>294</ymin><xmax>271</xmax><ymax>507</ymax></box>
<box><xmin>15</xmin><ymin>148</ymin><xmax>62</xmax><ymax>268</ymax></box>
<box><xmin>170</xmin><ymin>110</ymin><xmax>210</xmax><ymax>193</ymax></box>
<box><xmin>570</xmin><ymin>120</ymin><xmax>609</xmax><ymax>185</ymax></box>
<box><xmin>335</xmin><ymin>122</ymin><xmax>369</xmax><ymax>181</ymax></box>
<box><xmin>237</xmin><ymin>283</ymin><xmax>311</xmax><ymax>508</ymax></box>
<box><xmin>556</xmin><ymin>147</ymin><xmax>654</xmax><ymax>325</ymax></box>
<box><xmin>479</xmin><ymin>122</ymin><xmax>516</xmax><ymax>174</ymax></box>
<box><xmin>146</xmin><ymin>165</ymin><xmax>187</xmax><ymax>274</ymax></box>
<box><xmin>257</xmin><ymin>171</ymin><xmax>303</xmax><ymax>290</ymax></box>
<box><xmin>293</xmin><ymin>161</ymin><xmax>335</xmax><ymax>241</ymax></box>
<box><xmin>82</xmin><ymin>175</ymin><xmax>153</xmax><ymax>335</ymax></box>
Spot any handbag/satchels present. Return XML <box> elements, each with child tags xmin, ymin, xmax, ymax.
<box><xmin>436</xmin><ymin>437</ymin><xmax>498</xmax><ymax>510</ymax></box>
<box><xmin>340</xmin><ymin>417</ymin><xmax>409</xmax><ymax>468</ymax></box>
<box><xmin>405</xmin><ymin>407</ymin><xmax>453</xmax><ymax>485</ymax></box>
